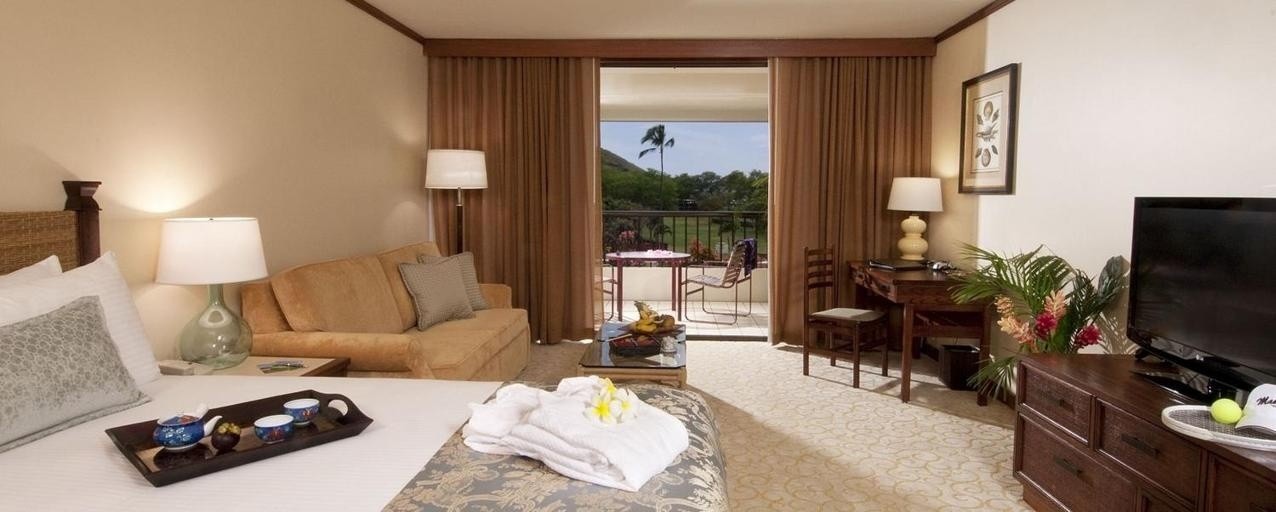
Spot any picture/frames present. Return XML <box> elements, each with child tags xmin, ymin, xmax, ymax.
<box><xmin>958</xmin><ymin>62</ymin><xmax>1017</xmax><ymax>195</ymax></box>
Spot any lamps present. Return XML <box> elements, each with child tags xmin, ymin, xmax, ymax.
<box><xmin>155</xmin><ymin>217</ymin><xmax>269</xmax><ymax>370</ymax></box>
<box><xmin>424</xmin><ymin>149</ymin><xmax>489</xmax><ymax>253</ymax></box>
<box><xmin>887</xmin><ymin>176</ymin><xmax>944</xmax><ymax>260</ymax></box>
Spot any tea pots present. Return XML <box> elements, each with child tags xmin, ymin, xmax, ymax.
<box><xmin>153</xmin><ymin>408</ymin><xmax>223</xmax><ymax>451</ymax></box>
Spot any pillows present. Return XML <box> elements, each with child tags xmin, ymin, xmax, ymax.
<box><xmin>0</xmin><ymin>255</ymin><xmax>169</xmax><ymax>442</ymax></box>
<box><xmin>397</xmin><ymin>251</ymin><xmax>488</xmax><ymax>333</ymax></box>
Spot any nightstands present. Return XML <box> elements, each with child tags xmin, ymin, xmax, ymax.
<box><xmin>199</xmin><ymin>356</ymin><xmax>351</xmax><ymax>376</ymax></box>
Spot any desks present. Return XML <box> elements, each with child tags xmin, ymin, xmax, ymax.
<box><xmin>606</xmin><ymin>250</ymin><xmax>691</xmax><ymax>321</ymax></box>
<box><xmin>851</xmin><ymin>261</ymin><xmax>995</xmax><ymax>407</ymax></box>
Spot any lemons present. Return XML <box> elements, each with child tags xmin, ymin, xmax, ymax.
<box><xmin>1210</xmin><ymin>398</ymin><xmax>1243</xmax><ymax>426</ymax></box>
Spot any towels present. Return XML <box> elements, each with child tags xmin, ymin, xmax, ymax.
<box><xmin>462</xmin><ymin>375</ymin><xmax>690</xmax><ymax>491</ymax></box>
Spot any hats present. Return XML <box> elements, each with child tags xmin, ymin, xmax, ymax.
<box><xmin>1235</xmin><ymin>383</ymin><xmax>1276</xmax><ymax>436</ymax></box>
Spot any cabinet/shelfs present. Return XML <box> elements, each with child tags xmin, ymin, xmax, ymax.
<box><xmin>1012</xmin><ymin>353</ymin><xmax>1276</xmax><ymax>512</ymax></box>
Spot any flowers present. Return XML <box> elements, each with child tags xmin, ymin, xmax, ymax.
<box><xmin>617</xmin><ymin>231</ymin><xmax>635</xmax><ymax>241</ymax></box>
<box><xmin>946</xmin><ymin>241</ymin><xmax>1125</xmax><ymax>402</ymax></box>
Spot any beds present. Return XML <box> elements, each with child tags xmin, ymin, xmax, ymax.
<box><xmin>0</xmin><ymin>180</ymin><xmax>727</xmax><ymax>511</ymax></box>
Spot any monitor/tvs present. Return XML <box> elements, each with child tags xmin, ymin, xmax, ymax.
<box><xmin>1126</xmin><ymin>197</ymin><xmax>1275</xmax><ymax>403</ymax></box>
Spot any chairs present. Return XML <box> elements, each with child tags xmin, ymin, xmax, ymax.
<box><xmin>685</xmin><ymin>239</ymin><xmax>756</xmax><ymax>325</ymax></box>
<box><xmin>593</xmin><ymin>264</ymin><xmax>614</xmax><ymax>321</ymax></box>
<box><xmin>803</xmin><ymin>244</ymin><xmax>890</xmax><ymax>389</ymax></box>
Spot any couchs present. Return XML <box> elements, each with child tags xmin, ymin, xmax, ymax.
<box><xmin>239</xmin><ymin>241</ymin><xmax>531</xmax><ymax>381</ymax></box>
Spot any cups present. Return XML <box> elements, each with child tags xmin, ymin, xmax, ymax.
<box><xmin>283</xmin><ymin>398</ymin><xmax>320</xmax><ymax>427</ymax></box>
<box><xmin>254</xmin><ymin>414</ymin><xmax>294</xmax><ymax>444</ymax></box>
